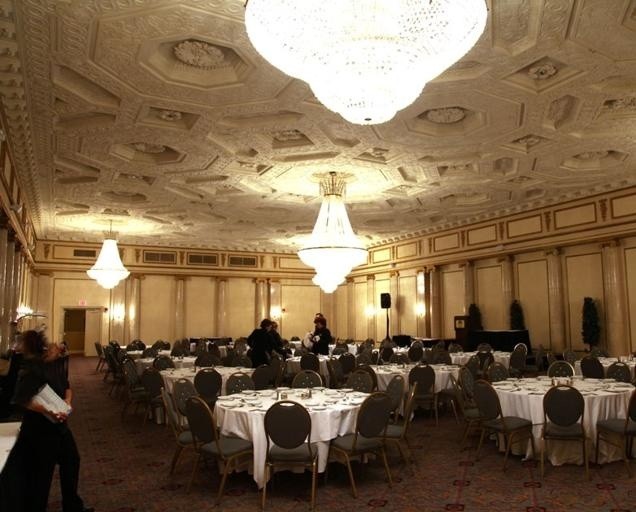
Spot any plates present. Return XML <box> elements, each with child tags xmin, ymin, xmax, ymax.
<box><xmin>612</xmin><ymin>384</ymin><xmax>630</xmax><ymax>387</ymax></box>
<box><xmin>221</xmin><ymin>401</ymin><xmax>241</xmax><ymax>406</ymax></box>
<box><xmin>520</xmin><ymin>378</ymin><xmax>536</xmax><ymax>382</ymax></box>
<box><xmin>497</xmin><ymin>386</ymin><xmax>511</xmax><ymax>390</ymax></box>
<box><xmin>492</xmin><ymin>381</ymin><xmax>513</xmax><ymax>385</ymax></box>
<box><xmin>256</xmin><ymin>390</ymin><xmax>275</xmax><ymax>395</ymax></box>
<box><xmin>603</xmin><ymin>388</ymin><xmax>629</xmax><ymax>392</ymax></box>
<box><xmin>528</xmin><ymin>391</ymin><xmax>545</xmax><ymax>395</ymax></box>
<box><xmin>242</xmin><ymin>389</ymin><xmax>253</xmax><ymax>394</ymax></box>
<box><xmin>324</xmin><ymin>392</ymin><xmax>337</xmax><ymax>394</ymax></box>
<box><xmin>246</xmin><ymin>401</ymin><xmax>262</xmax><ymax>405</ymax></box>
<box><xmin>217</xmin><ymin>396</ymin><xmax>231</xmax><ymax>399</ymax></box>
<box><xmin>312</xmin><ymin>387</ymin><xmax>325</xmax><ymax>389</ymax></box>
<box><xmin>230</xmin><ymin>394</ymin><xmax>244</xmax><ymax>398</ymax></box>
<box><xmin>277</xmin><ymin>387</ymin><xmax>290</xmax><ymax>389</ymax></box>
<box><xmin>337</xmin><ymin>389</ymin><xmax>353</xmax><ymax>392</ymax></box>
<box><xmin>324</xmin><ymin>400</ymin><xmax>339</xmax><ymax>403</ymax></box>
<box><xmin>245</xmin><ymin>396</ymin><xmax>258</xmax><ymax>399</ymax></box>
<box><xmin>311</xmin><ymin>407</ymin><xmax>326</xmax><ymax>410</ymax></box>
<box><xmin>352</xmin><ymin>398</ymin><xmax>364</xmax><ymax>404</ymax></box>
<box><xmin>330</xmin><ymin>396</ymin><xmax>343</xmax><ymax>398</ymax></box>
<box><xmin>507</xmin><ymin>377</ymin><xmax>516</xmax><ymax>380</ymax></box>
<box><xmin>584</xmin><ymin>378</ymin><xmax>599</xmax><ymax>382</ymax></box>
<box><xmin>305</xmin><ymin>403</ymin><xmax>319</xmax><ymax>406</ymax></box>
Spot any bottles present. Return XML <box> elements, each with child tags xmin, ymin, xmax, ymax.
<box><xmin>574</xmin><ymin>360</ymin><xmax>581</xmax><ymax>376</ymax></box>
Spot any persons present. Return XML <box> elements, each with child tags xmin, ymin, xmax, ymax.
<box><xmin>246</xmin><ymin>318</ymin><xmax>291</xmax><ymax>367</ymax></box>
<box><xmin>309</xmin><ymin>316</ymin><xmax>331</xmax><ymax>355</ymax></box>
<box><xmin>270</xmin><ymin>321</ymin><xmax>283</xmax><ymax>350</ymax></box>
<box><xmin>0</xmin><ymin>330</ymin><xmax>96</xmax><ymax>512</ymax></box>
<box><xmin>315</xmin><ymin>312</ymin><xmax>323</xmax><ymax>332</ymax></box>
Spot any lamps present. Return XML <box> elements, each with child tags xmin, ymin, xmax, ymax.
<box><xmin>86</xmin><ymin>220</ymin><xmax>132</xmax><ymax>288</ymax></box>
<box><xmin>244</xmin><ymin>0</ymin><xmax>487</xmax><ymax>125</ymax></box>
<box><xmin>297</xmin><ymin>171</ymin><xmax>368</xmax><ymax>293</ymax></box>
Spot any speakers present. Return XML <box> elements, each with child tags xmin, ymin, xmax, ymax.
<box><xmin>380</xmin><ymin>293</ymin><xmax>391</xmax><ymax>308</ymax></box>
